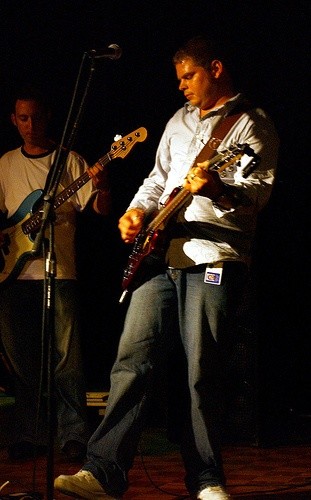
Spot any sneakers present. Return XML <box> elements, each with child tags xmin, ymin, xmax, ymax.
<box><xmin>11</xmin><ymin>441</ymin><xmax>46</xmax><ymax>463</ymax></box>
<box><xmin>62</xmin><ymin>440</ymin><xmax>87</xmax><ymax>462</ymax></box>
<box><xmin>190</xmin><ymin>485</ymin><xmax>232</xmax><ymax>500</ymax></box>
<box><xmin>54</xmin><ymin>469</ymin><xmax>122</xmax><ymax>500</ymax></box>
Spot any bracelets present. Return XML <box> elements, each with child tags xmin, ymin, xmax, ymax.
<box><xmin>212</xmin><ymin>183</ymin><xmax>228</xmax><ymax>204</ymax></box>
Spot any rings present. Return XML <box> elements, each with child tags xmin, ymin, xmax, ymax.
<box><xmin>133</xmin><ymin>215</ymin><xmax>142</xmax><ymax>221</ymax></box>
<box><xmin>191</xmin><ymin>173</ymin><xmax>196</xmax><ymax>179</ymax></box>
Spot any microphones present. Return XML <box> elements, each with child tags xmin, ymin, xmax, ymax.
<box><xmin>86</xmin><ymin>44</ymin><xmax>121</xmax><ymax>59</ymax></box>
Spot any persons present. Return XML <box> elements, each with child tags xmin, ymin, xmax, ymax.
<box><xmin>0</xmin><ymin>90</ymin><xmax>113</xmax><ymax>463</ymax></box>
<box><xmin>53</xmin><ymin>35</ymin><xmax>279</xmax><ymax>500</ymax></box>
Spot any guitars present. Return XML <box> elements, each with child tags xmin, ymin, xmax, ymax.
<box><xmin>118</xmin><ymin>141</ymin><xmax>255</xmax><ymax>289</ymax></box>
<box><xmin>0</xmin><ymin>126</ymin><xmax>148</xmax><ymax>287</ymax></box>
